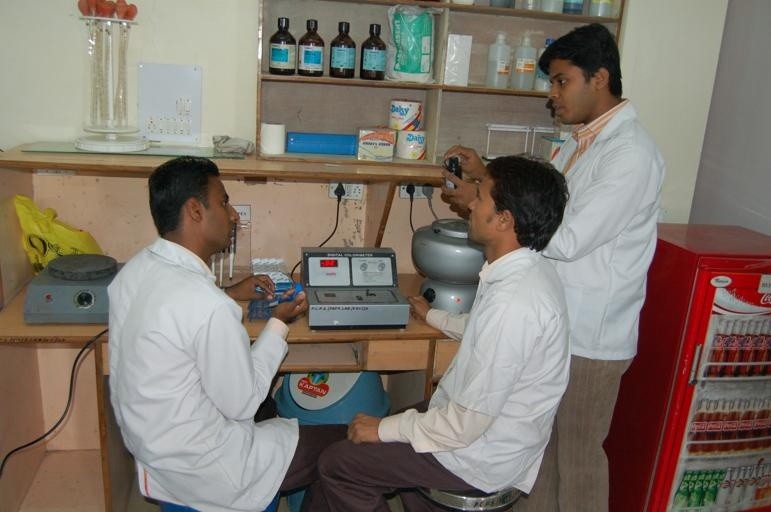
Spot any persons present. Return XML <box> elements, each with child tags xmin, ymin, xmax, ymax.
<box><xmin>439</xmin><ymin>21</ymin><xmax>667</xmax><ymax>512</ymax></box>
<box><xmin>296</xmin><ymin>152</ymin><xmax>573</xmax><ymax>512</ymax></box>
<box><xmin>106</xmin><ymin>155</ymin><xmax>348</xmax><ymax>512</ymax></box>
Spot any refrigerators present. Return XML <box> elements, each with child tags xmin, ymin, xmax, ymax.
<box><xmin>600</xmin><ymin>223</ymin><xmax>771</xmax><ymax>512</ymax></box>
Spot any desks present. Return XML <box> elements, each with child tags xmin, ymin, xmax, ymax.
<box><xmin>0</xmin><ymin>140</ymin><xmax>460</xmax><ymax>512</ymax></box>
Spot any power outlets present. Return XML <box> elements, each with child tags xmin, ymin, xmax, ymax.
<box><xmin>329</xmin><ymin>182</ymin><xmax>363</xmax><ymax>200</ymax></box>
<box><xmin>399</xmin><ymin>182</ymin><xmax>434</xmax><ymax>199</ymax></box>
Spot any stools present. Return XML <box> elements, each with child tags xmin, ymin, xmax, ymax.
<box><xmin>160</xmin><ymin>493</ymin><xmax>287</xmax><ymax>511</ymax></box>
<box><xmin>417</xmin><ymin>485</ymin><xmax>523</xmax><ymax>512</ymax></box>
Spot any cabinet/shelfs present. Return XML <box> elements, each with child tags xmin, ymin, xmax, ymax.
<box><xmin>257</xmin><ymin>1</ymin><xmax>627</xmax><ymax>169</ymax></box>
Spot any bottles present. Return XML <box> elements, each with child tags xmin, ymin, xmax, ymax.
<box><xmin>485</xmin><ymin>30</ymin><xmax>557</xmax><ymax>92</ymax></box>
<box><xmin>443</xmin><ymin>155</ymin><xmax>462</xmax><ymax>191</ymax></box>
<box><xmin>706</xmin><ymin>319</ymin><xmax>771</xmax><ymax>376</ymax></box>
<box><xmin>328</xmin><ymin>21</ymin><xmax>357</xmax><ymax>77</ymax></box>
<box><xmin>269</xmin><ymin>16</ymin><xmax>325</xmax><ymax>77</ymax></box>
<box><xmin>686</xmin><ymin>396</ymin><xmax>770</xmax><ymax>455</ymax></box>
<box><xmin>673</xmin><ymin>463</ymin><xmax>770</xmax><ymax>512</ymax></box>
<box><xmin>359</xmin><ymin>23</ymin><xmax>387</xmax><ymax>80</ymax></box>
